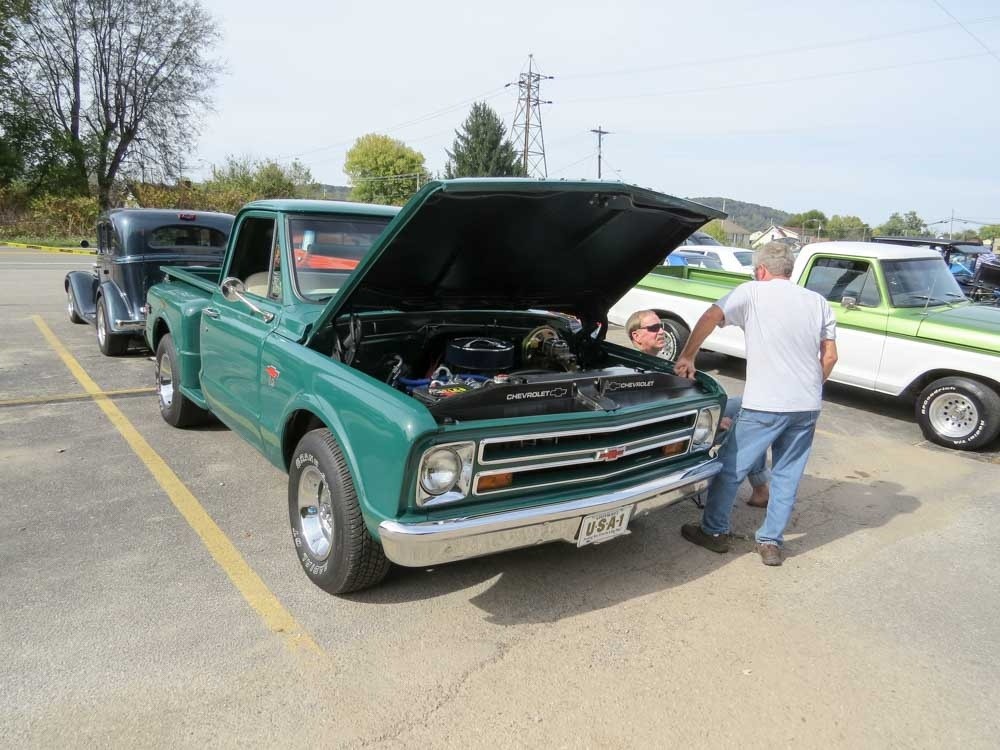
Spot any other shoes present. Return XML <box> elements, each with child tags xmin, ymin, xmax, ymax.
<box><xmin>747</xmin><ymin>487</ymin><xmax>773</xmax><ymax>508</ymax></box>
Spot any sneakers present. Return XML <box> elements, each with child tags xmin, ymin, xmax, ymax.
<box><xmin>756</xmin><ymin>542</ymin><xmax>783</xmax><ymax>565</ymax></box>
<box><xmin>682</xmin><ymin>524</ymin><xmax>729</xmax><ymax>554</ymax></box>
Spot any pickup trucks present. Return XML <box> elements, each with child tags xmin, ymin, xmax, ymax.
<box><xmin>146</xmin><ymin>171</ymin><xmax>730</xmax><ymax>597</ymax></box>
<box><xmin>604</xmin><ymin>239</ymin><xmax>1000</xmax><ymax>453</ymax></box>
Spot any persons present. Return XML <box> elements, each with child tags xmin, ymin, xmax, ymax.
<box><xmin>260</xmin><ymin>245</ymin><xmax>282</xmax><ymax>273</ymax></box>
<box><xmin>674</xmin><ymin>240</ymin><xmax>837</xmax><ymax>566</ymax></box>
<box><xmin>627</xmin><ymin>309</ymin><xmax>772</xmax><ymax>509</ymax></box>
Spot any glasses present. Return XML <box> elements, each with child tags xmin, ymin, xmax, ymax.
<box><xmin>751</xmin><ymin>266</ymin><xmax>761</xmax><ymax>279</ymax></box>
<box><xmin>638</xmin><ymin>321</ymin><xmax>664</xmax><ymax>332</ymax></box>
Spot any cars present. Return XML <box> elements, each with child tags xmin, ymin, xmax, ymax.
<box><xmin>63</xmin><ymin>207</ymin><xmax>238</xmax><ymax>358</ymax></box>
<box><xmin>597</xmin><ymin>228</ymin><xmax>1000</xmax><ymax>310</ymax></box>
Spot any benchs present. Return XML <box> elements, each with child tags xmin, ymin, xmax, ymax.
<box><xmin>246</xmin><ymin>273</ymin><xmax>352</xmax><ymax>298</ymax></box>
